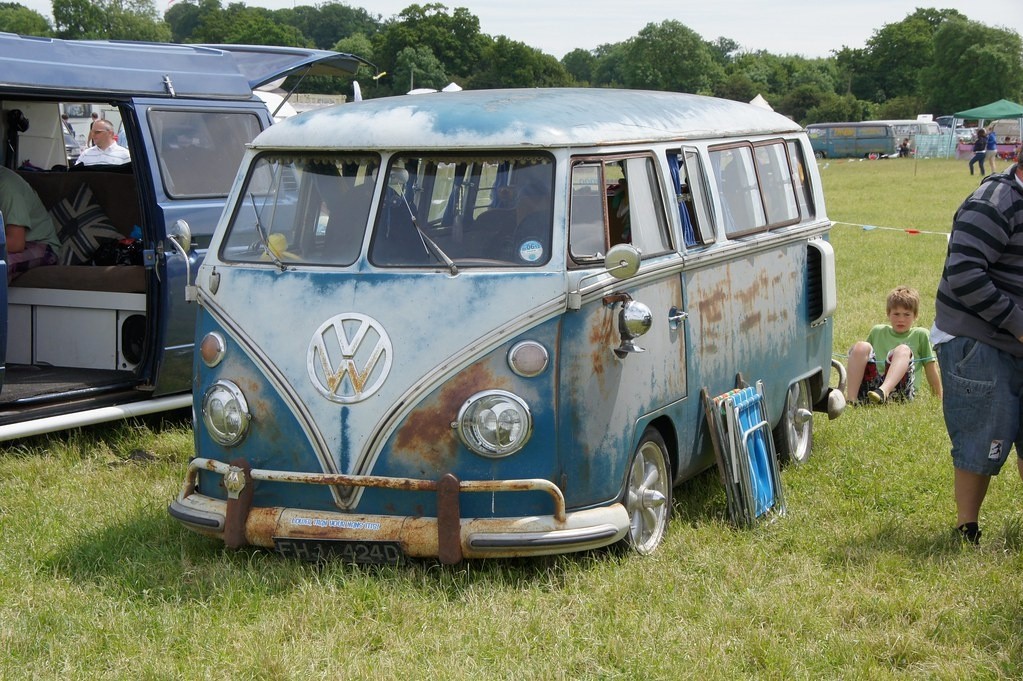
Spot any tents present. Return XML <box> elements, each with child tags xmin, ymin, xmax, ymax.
<box><xmin>946</xmin><ymin>99</ymin><xmax>1023</xmax><ymax>160</ymax></box>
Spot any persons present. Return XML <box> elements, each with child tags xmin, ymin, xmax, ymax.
<box><xmin>846</xmin><ymin>285</ymin><xmax>942</xmax><ymax>405</ymax></box>
<box><xmin>87</xmin><ymin>113</ymin><xmax>98</xmax><ymax>148</ymax></box>
<box><xmin>985</xmin><ymin>126</ymin><xmax>997</xmax><ymax>174</ymax></box>
<box><xmin>956</xmin><ymin>138</ymin><xmax>965</xmax><ymax>148</ymax></box>
<box><xmin>61</xmin><ymin>114</ymin><xmax>75</xmax><ymax>136</ymax></box>
<box><xmin>928</xmin><ymin>143</ymin><xmax>1023</xmax><ymax>544</ymax></box>
<box><xmin>899</xmin><ymin>139</ymin><xmax>910</xmax><ymax>157</ymax></box>
<box><xmin>322</xmin><ymin>181</ymin><xmax>415</xmax><ymax>261</ymax></box>
<box><xmin>469</xmin><ymin>179</ymin><xmax>551</xmax><ymax>236</ymax></box>
<box><xmin>74</xmin><ymin>120</ymin><xmax>131</xmax><ymax>165</ymax></box>
<box><xmin>0</xmin><ymin>166</ymin><xmax>62</xmax><ymax>287</ymax></box>
<box><xmin>1005</xmin><ymin>137</ymin><xmax>1010</xmax><ymax>144</ymax></box>
<box><xmin>969</xmin><ymin>130</ymin><xmax>988</xmax><ymax>177</ymax></box>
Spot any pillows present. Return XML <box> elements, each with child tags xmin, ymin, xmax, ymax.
<box><xmin>48</xmin><ymin>180</ymin><xmax>126</xmax><ymax>265</ymax></box>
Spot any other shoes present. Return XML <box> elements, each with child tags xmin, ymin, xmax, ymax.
<box><xmin>955</xmin><ymin>522</ymin><xmax>982</xmax><ymax>549</ymax></box>
<box><xmin>846</xmin><ymin>400</ymin><xmax>859</xmax><ymax>408</ymax></box>
<box><xmin>867</xmin><ymin>388</ymin><xmax>884</xmax><ymax>405</ymax></box>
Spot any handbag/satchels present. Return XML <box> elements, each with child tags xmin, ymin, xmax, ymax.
<box><xmin>79</xmin><ymin>239</ymin><xmax>144</xmax><ymax>266</ymax></box>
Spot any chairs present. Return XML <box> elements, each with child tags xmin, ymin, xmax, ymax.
<box><xmin>323</xmin><ymin>183</ymin><xmax>426</xmax><ymax>264</ymax></box>
<box><xmin>511</xmin><ymin>209</ymin><xmax>552</xmax><ymax>264</ymax></box>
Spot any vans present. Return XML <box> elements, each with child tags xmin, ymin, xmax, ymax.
<box><xmin>936</xmin><ymin>115</ymin><xmax>993</xmax><ymax>145</ymax></box>
<box><xmin>804</xmin><ymin>122</ymin><xmax>897</xmax><ymax>161</ymax></box>
<box><xmin>0</xmin><ymin>35</ymin><xmax>383</xmax><ymax>449</ymax></box>
<box><xmin>167</xmin><ymin>87</ymin><xmax>849</xmax><ymax>577</ymax></box>
<box><xmin>861</xmin><ymin>119</ymin><xmax>942</xmax><ymax>157</ymax></box>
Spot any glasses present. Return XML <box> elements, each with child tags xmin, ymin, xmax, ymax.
<box><xmin>91</xmin><ymin>130</ymin><xmax>110</xmax><ymax>134</ymax></box>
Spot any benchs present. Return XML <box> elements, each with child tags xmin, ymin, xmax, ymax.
<box><xmin>12</xmin><ymin>169</ymin><xmax>146</xmax><ymax>293</ymax></box>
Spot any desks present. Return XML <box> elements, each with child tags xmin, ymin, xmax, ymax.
<box><xmin>957</xmin><ymin>143</ymin><xmax>1020</xmax><ymax>162</ymax></box>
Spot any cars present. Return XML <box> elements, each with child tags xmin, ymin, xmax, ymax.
<box><xmin>61</xmin><ymin>118</ymin><xmax>80</xmax><ymax>167</ymax></box>
<box><xmin>115</xmin><ymin>118</ymin><xmax>128</xmax><ymax>150</ymax></box>
